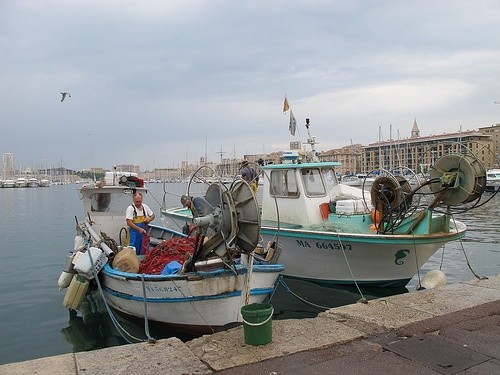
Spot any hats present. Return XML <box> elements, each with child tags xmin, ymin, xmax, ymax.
<box><xmin>181</xmin><ymin>194</ymin><xmax>190</xmax><ymax>208</ymax></box>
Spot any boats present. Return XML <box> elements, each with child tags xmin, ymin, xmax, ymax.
<box><xmin>160</xmin><ymin>116</ymin><xmax>500</xmax><ymax>292</ymax></box>
<box><xmin>56</xmin><ymin>160</ymin><xmax>284</xmax><ymax>338</ymax></box>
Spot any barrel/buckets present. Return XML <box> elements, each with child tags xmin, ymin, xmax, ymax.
<box><xmin>111</xmin><ymin>246</ymin><xmax>139</xmax><ymax>274</ymax></box>
<box><xmin>241</xmin><ymin>303</ymin><xmax>275</xmax><ymax>346</ymax></box>
<box><xmin>57</xmin><ymin>237</ymin><xmax>109</xmax><ymax>318</ymax></box>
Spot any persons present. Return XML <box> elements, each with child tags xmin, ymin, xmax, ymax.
<box><xmin>181</xmin><ymin>195</ymin><xmax>210</xmax><ymax>237</ymax></box>
<box><xmin>241</xmin><ymin>161</ymin><xmax>260</xmax><ymax>191</ymax></box>
<box><xmin>127</xmin><ymin>193</ymin><xmax>155</xmax><ymax>255</ymax></box>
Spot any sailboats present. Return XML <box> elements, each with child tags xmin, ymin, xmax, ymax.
<box><xmin>141</xmin><ymin>134</ymin><xmax>264</xmax><ymax>185</ymax></box>
<box><xmin>0</xmin><ymin>153</ymin><xmax>93</xmax><ymax>188</ymax></box>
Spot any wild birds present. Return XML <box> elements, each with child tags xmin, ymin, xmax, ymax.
<box><xmin>58</xmin><ymin>91</ymin><xmax>72</xmax><ymax>102</ymax></box>
